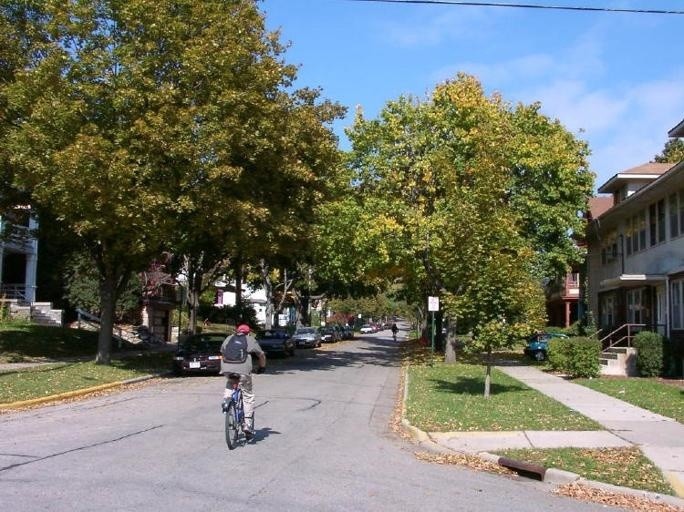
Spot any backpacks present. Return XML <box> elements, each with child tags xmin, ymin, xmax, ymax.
<box><xmin>223</xmin><ymin>333</ymin><xmax>247</xmax><ymax>364</ymax></box>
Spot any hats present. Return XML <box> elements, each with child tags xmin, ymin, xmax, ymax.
<box><xmin>236</xmin><ymin>324</ymin><xmax>251</xmax><ymax>332</ymax></box>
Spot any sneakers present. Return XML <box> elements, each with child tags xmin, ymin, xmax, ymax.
<box><xmin>243</xmin><ymin>423</ymin><xmax>253</xmax><ymax>434</ymax></box>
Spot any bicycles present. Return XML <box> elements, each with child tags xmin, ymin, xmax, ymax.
<box><xmin>221</xmin><ymin>367</ymin><xmax>264</xmax><ymax>451</ymax></box>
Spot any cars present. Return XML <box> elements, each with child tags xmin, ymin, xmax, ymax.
<box><xmin>359</xmin><ymin>319</ymin><xmax>393</xmax><ymax>335</ymax></box>
<box><xmin>523</xmin><ymin>332</ymin><xmax>572</xmax><ymax>362</ymax></box>
<box><xmin>316</xmin><ymin>326</ymin><xmax>355</xmax><ymax>343</ymax></box>
<box><xmin>174</xmin><ymin>331</ymin><xmax>232</xmax><ymax>375</ymax></box>
<box><xmin>253</xmin><ymin>328</ymin><xmax>295</xmax><ymax>356</ymax></box>
<box><xmin>292</xmin><ymin>327</ymin><xmax>322</xmax><ymax>348</ymax></box>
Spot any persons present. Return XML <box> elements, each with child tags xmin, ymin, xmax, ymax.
<box><xmin>392</xmin><ymin>323</ymin><xmax>399</xmax><ymax>336</ymax></box>
<box><xmin>219</xmin><ymin>324</ymin><xmax>266</xmax><ymax>437</ymax></box>
